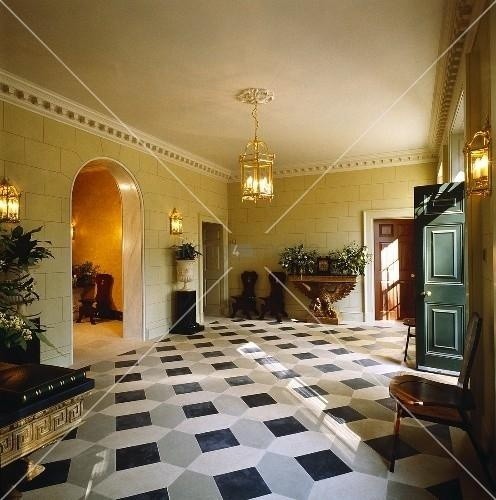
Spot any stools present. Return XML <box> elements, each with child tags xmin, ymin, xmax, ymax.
<box><xmin>401</xmin><ymin>317</ymin><xmax>417</xmax><ymax>362</ymax></box>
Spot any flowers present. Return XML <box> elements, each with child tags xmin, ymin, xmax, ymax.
<box><xmin>0</xmin><ymin>310</ymin><xmax>59</xmax><ymax>354</ymax></box>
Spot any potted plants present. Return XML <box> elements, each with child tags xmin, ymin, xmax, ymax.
<box><xmin>329</xmin><ymin>240</ymin><xmax>372</xmax><ymax>276</ymax></box>
<box><xmin>71</xmin><ymin>260</ymin><xmax>103</xmax><ymax>301</ymax></box>
<box><xmin>0</xmin><ymin>221</ymin><xmax>56</xmax><ymax>321</ymax></box>
<box><xmin>171</xmin><ymin>239</ymin><xmax>204</xmax><ymax>291</ymax></box>
<box><xmin>278</xmin><ymin>243</ymin><xmax>320</xmax><ymax>275</ymax></box>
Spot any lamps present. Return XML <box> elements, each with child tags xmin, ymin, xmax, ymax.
<box><xmin>461</xmin><ymin>116</ymin><xmax>494</xmax><ymax>201</ymax></box>
<box><xmin>169</xmin><ymin>207</ymin><xmax>183</xmax><ymax>235</ymax></box>
<box><xmin>0</xmin><ymin>174</ymin><xmax>24</xmax><ymax>227</ymax></box>
<box><xmin>235</xmin><ymin>85</ymin><xmax>279</xmax><ymax>207</ymax></box>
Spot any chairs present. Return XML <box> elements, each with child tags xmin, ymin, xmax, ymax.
<box><xmin>91</xmin><ymin>274</ymin><xmax>114</xmax><ymax>322</ymax></box>
<box><xmin>227</xmin><ymin>268</ymin><xmax>289</xmax><ymax>323</ymax></box>
<box><xmin>386</xmin><ymin>311</ymin><xmax>491</xmax><ymax>480</ymax></box>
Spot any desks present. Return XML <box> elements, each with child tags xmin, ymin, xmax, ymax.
<box><xmin>286</xmin><ymin>273</ymin><xmax>359</xmax><ymax>326</ymax></box>
<box><xmin>1</xmin><ymin>359</ymin><xmax>97</xmax><ymax>499</ymax></box>
